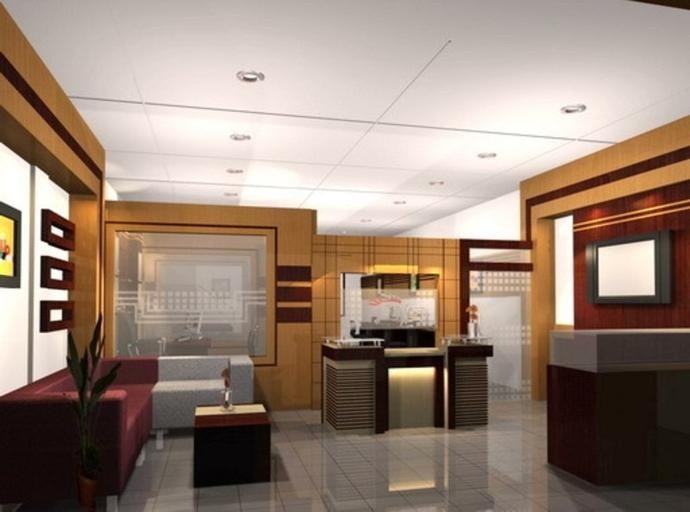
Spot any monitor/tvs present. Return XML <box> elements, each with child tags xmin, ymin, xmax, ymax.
<box><xmin>585</xmin><ymin>228</ymin><xmax>672</xmax><ymax>305</ymax></box>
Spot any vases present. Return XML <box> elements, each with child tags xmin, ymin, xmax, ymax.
<box><xmin>467</xmin><ymin>318</ymin><xmax>481</xmax><ymax>340</ymax></box>
<box><xmin>220</xmin><ymin>388</ymin><xmax>234</xmax><ymax>412</ymax></box>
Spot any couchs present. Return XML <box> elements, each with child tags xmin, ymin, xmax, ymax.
<box><xmin>1</xmin><ymin>356</ymin><xmax>160</xmax><ymax>512</ymax></box>
<box><xmin>151</xmin><ymin>355</ymin><xmax>254</xmax><ymax>437</ymax></box>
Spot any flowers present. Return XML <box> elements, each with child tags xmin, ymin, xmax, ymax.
<box><xmin>221</xmin><ymin>368</ymin><xmax>232</xmax><ymax>389</ymax></box>
<box><xmin>465</xmin><ymin>305</ymin><xmax>481</xmax><ymax>322</ymax></box>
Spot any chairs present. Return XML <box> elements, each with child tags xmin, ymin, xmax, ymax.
<box><xmin>115</xmin><ymin>309</ymin><xmax>258</xmax><ymax>357</ymax></box>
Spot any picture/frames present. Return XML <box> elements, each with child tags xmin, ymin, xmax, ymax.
<box><xmin>0</xmin><ymin>199</ymin><xmax>22</xmax><ymax>287</ymax></box>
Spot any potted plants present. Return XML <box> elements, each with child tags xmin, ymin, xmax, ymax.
<box><xmin>62</xmin><ymin>311</ymin><xmax>122</xmax><ymax>511</ymax></box>
